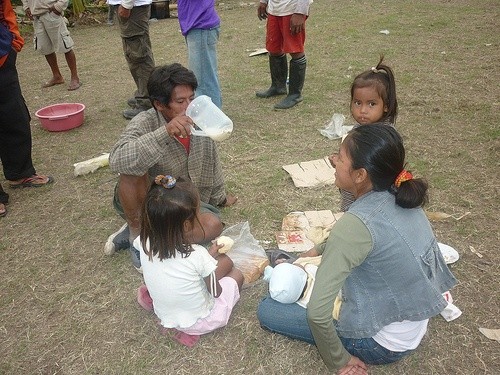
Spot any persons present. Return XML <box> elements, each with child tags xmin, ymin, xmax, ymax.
<box><xmin>21</xmin><ymin>0</ymin><xmax>80</xmax><ymax>91</ymax></box>
<box><xmin>107</xmin><ymin>0</ymin><xmax>157</xmax><ymax>122</ymax></box>
<box><xmin>251</xmin><ymin>122</ymin><xmax>457</xmax><ymax>375</ymax></box>
<box><xmin>339</xmin><ymin>57</ymin><xmax>399</xmax><ymax>216</ymax></box>
<box><xmin>102</xmin><ymin>62</ymin><xmax>237</xmax><ymax>276</ymax></box>
<box><xmin>176</xmin><ymin>1</ymin><xmax>223</xmax><ymax>110</ymax></box>
<box><xmin>133</xmin><ymin>177</ymin><xmax>246</xmax><ymax>347</ymax></box>
<box><xmin>0</xmin><ymin>0</ymin><xmax>55</xmax><ymax>218</ymax></box>
<box><xmin>255</xmin><ymin>0</ymin><xmax>310</xmax><ymax>111</ymax></box>
<box><xmin>263</xmin><ymin>255</ymin><xmax>342</xmax><ymax>320</ymax></box>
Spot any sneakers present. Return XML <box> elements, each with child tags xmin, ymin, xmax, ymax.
<box><xmin>130</xmin><ymin>246</ymin><xmax>142</xmax><ymax>273</ymax></box>
<box><xmin>104</xmin><ymin>222</ymin><xmax>130</xmax><ymax>256</ymax></box>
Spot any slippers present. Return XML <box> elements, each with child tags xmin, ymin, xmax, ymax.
<box><xmin>0</xmin><ymin>205</ymin><xmax>8</xmax><ymax>217</ymax></box>
<box><xmin>9</xmin><ymin>176</ymin><xmax>54</xmax><ymax>189</ymax></box>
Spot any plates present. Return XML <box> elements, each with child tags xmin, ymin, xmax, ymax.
<box><xmin>436</xmin><ymin>242</ymin><xmax>459</xmax><ymax>265</ymax></box>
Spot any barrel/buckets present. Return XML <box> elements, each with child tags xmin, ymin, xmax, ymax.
<box><xmin>151</xmin><ymin>1</ymin><xmax>169</xmax><ymax>19</ymax></box>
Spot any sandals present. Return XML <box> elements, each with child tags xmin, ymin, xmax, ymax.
<box><xmin>266</xmin><ymin>248</ymin><xmax>296</xmax><ymax>264</ymax></box>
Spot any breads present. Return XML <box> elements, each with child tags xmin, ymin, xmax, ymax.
<box><xmin>217</xmin><ymin>236</ymin><xmax>233</xmax><ymax>253</ymax></box>
<box><xmin>243</xmin><ymin>258</ymin><xmax>269</xmax><ymax>283</ymax></box>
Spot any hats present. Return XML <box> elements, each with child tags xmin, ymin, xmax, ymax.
<box><xmin>264</xmin><ymin>262</ymin><xmax>308</xmax><ymax>303</ymax></box>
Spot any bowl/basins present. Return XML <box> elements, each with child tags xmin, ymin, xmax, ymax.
<box><xmin>35</xmin><ymin>102</ymin><xmax>85</xmax><ymax>132</ymax></box>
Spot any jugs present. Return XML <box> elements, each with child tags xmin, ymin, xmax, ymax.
<box><xmin>185</xmin><ymin>95</ymin><xmax>233</xmax><ymax>142</ymax></box>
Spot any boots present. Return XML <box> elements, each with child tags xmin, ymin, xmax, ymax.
<box><xmin>256</xmin><ymin>53</ymin><xmax>287</xmax><ymax>98</ymax></box>
<box><xmin>274</xmin><ymin>55</ymin><xmax>306</xmax><ymax>111</ymax></box>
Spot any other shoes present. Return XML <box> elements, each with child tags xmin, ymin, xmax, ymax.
<box><xmin>123</xmin><ymin>108</ymin><xmax>143</xmax><ymax>118</ymax></box>
<box><xmin>138</xmin><ymin>285</ymin><xmax>153</xmax><ymax>311</ymax></box>
<box><xmin>163</xmin><ymin>326</ymin><xmax>199</xmax><ymax>346</ymax></box>
<box><xmin>127</xmin><ymin>99</ymin><xmax>135</xmax><ymax>107</ymax></box>
